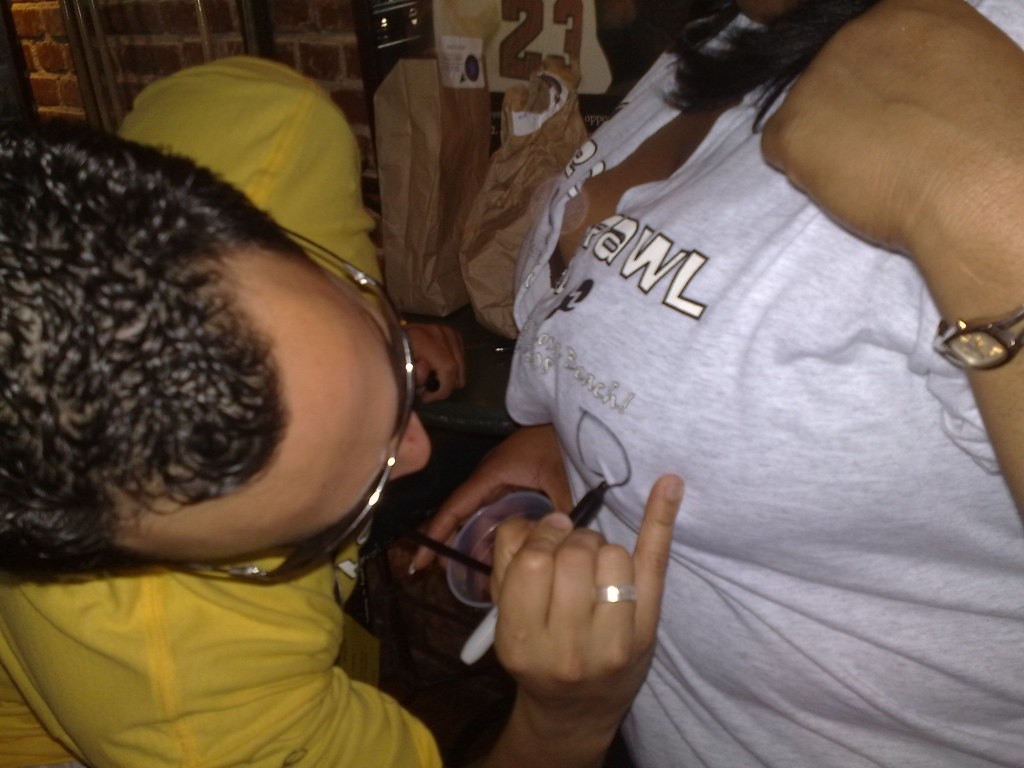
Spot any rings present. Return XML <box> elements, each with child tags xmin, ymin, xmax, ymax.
<box><xmin>591</xmin><ymin>584</ymin><xmax>639</xmax><ymax>606</ymax></box>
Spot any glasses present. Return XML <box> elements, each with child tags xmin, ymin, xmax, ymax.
<box><xmin>175</xmin><ymin>219</ymin><xmax>419</xmax><ymax>585</ymax></box>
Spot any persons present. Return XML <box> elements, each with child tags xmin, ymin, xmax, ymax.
<box><xmin>1</xmin><ymin>56</ymin><xmax>684</xmax><ymax>768</ymax></box>
<box><xmin>407</xmin><ymin>0</ymin><xmax>1024</xmax><ymax>768</ymax></box>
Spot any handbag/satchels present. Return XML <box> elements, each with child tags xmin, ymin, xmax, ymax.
<box><xmin>374</xmin><ymin>57</ymin><xmax>487</xmax><ymax>316</ymax></box>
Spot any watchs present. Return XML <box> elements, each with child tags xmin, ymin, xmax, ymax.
<box><xmin>932</xmin><ymin>307</ymin><xmax>1024</xmax><ymax>372</ymax></box>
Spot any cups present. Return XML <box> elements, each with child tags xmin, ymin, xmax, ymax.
<box><xmin>446</xmin><ymin>492</ymin><xmax>553</xmax><ymax>607</ymax></box>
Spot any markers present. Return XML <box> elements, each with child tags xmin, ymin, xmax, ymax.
<box><xmin>460</xmin><ymin>480</ymin><xmax>611</xmax><ymax>666</ymax></box>
